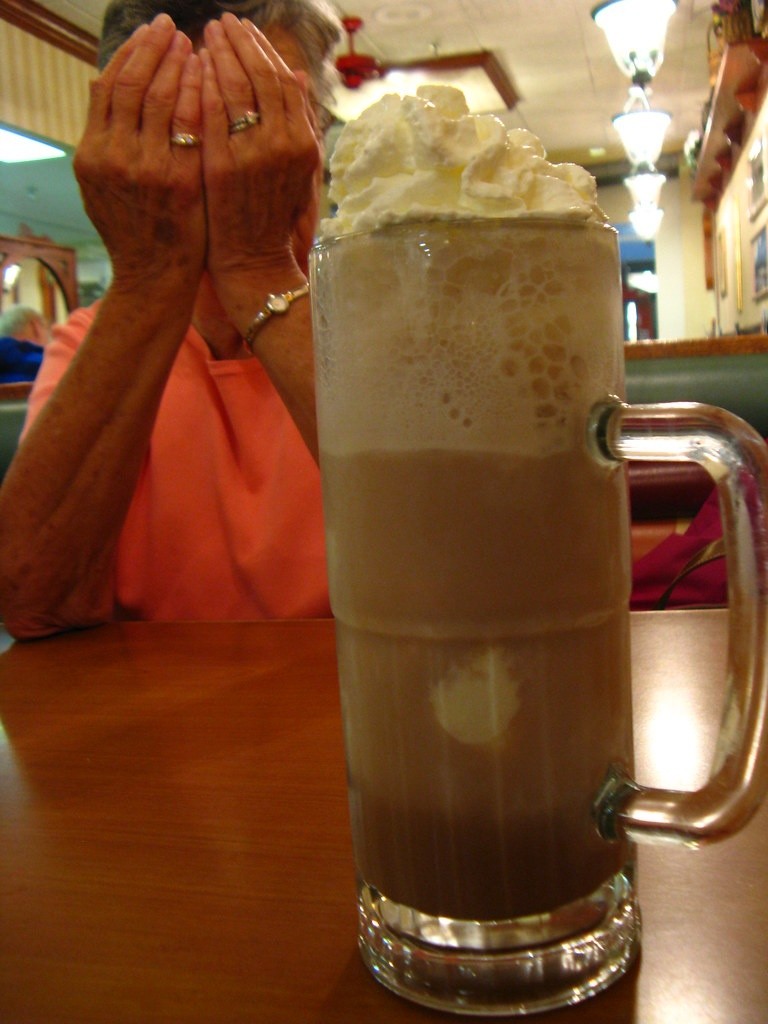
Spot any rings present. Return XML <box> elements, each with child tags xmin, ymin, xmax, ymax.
<box><xmin>229</xmin><ymin>111</ymin><xmax>259</xmax><ymax>134</ymax></box>
<box><xmin>172</xmin><ymin>134</ymin><xmax>199</xmax><ymax>145</ymax></box>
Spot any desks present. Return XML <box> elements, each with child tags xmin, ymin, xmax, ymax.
<box><xmin>0</xmin><ymin>606</ymin><xmax>768</xmax><ymax>1024</ymax></box>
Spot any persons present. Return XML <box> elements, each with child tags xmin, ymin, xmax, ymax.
<box><xmin>0</xmin><ymin>0</ymin><xmax>389</xmax><ymax>640</ymax></box>
<box><xmin>0</xmin><ymin>304</ymin><xmax>46</xmax><ymax>385</ymax></box>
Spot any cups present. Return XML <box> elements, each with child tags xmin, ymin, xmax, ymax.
<box><xmin>303</xmin><ymin>218</ymin><xmax>768</xmax><ymax>1016</ymax></box>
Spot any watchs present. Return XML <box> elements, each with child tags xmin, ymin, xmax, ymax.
<box><xmin>244</xmin><ymin>284</ymin><xmax>311</xmax><ymax>345</ymax></box>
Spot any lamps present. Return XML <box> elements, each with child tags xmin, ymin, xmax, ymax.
<box><xmin>594</xmin><ymin>0</ymin><xmax>681</xmax><ymax>87</ymax></box>
<box><xmin>624</xmin><ymin>164</ymin><xmax>665</xmax><ymax>242</ymax></box>
<box><xmin>612</xmin><ymin>89</ymin><xmax>671</xmax><ymax>169</ymax></box>
